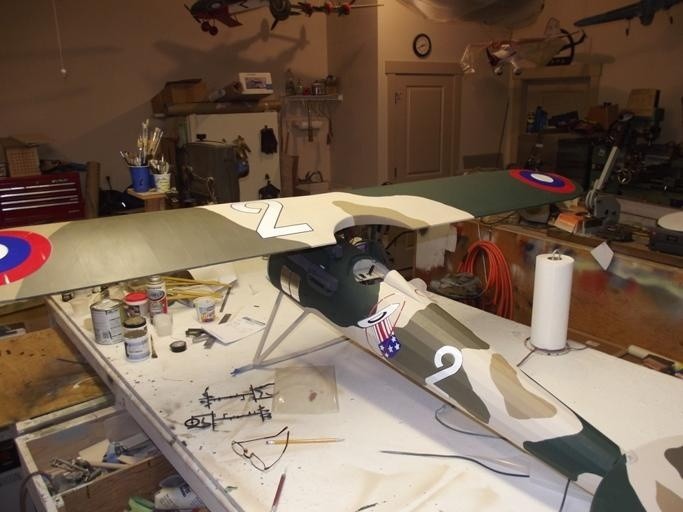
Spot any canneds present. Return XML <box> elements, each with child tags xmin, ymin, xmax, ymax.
<box><xmin>90</xmin><ymin>298</ymin><xmax>125</xmax><ymax>345</ymax></box>
<box><xmin>124</xmin><ymin>330</ymin><xmax>151</xmax><ymax>361</ymax></box>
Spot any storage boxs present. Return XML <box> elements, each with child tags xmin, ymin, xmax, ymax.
<box><xmin>151</xmin><ymin>81</ymin><xmax>209</xmax><ymax>113</ymax></box>
<box><xmin>590</xmin><ymin>104</ymin><xmax>618</xmax><ymax>132</ymax></box>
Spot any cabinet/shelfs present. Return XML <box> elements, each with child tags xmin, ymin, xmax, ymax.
<box><xmin>518</xmin><ymin>133</ymin><xmax>593</xmax><ymax>193</ymax></box>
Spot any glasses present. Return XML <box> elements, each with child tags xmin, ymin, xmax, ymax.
<box><xmin>231</xmin><ymin>426</ymin><xmax>290</xmax><ymax>471</ymax></box>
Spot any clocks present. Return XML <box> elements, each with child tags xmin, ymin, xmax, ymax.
<box><xmin>413</xmin><ymin>34</ymin><xmax>431</xmax><ymax>57</ymax></box>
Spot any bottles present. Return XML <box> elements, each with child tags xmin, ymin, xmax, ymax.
<box><xmin>287</xmin><ymin>77</ymin><xmax>295</xmax><ymax>95</ymax></box>
<box><xmin>122</xmin><ymin>316</ymin><xmax>150</xmax><ymax>362</ymax></box>
<box><xmin>312</xmin><ymin>80</ymin><xmax>321</xmax><ymax>95</ymax></box>
<box><xmin>295</xmin><ymin>80</ymin><xmax>303</xmax><ymax>95</ymax></box>
<box><xmin>149</xmin><ymin>290</ymin><xmax>166</xmax><ymax>325</ymax></box>
<box><xmin>147</xmin><ymin>275</ymin><xmax>167</xmax><ymax>307</ymax></box>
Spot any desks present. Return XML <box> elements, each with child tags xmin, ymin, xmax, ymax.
<box><xmin>1</xmin><ymin>254</ymin><xmax>683</xmax><ymax>512</ymax></box>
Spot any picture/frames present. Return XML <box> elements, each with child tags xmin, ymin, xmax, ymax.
<box><xmin>238</xmin><ymin>72</ymin><xmax>274</xmax><ymax>95</ymax></box>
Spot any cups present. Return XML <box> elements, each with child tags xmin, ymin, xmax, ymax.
<box><xmin>128</xmin><ymin>165</ymin><xmax>148</xmax><ymax>192</ymax></box>
<box><xmin>193</xmin><ymin>297</ymin><xmax>215</xmax><ymax>322</ymax></box>
<box><xmin>152</xmin><ymin>173</ymin><xmax>171</xmax><ymax>194</ymax></box>
<box><xmin>153</xmin><ymin>313</ymin><xmax>174</xmax><ymax>338</ymax></box>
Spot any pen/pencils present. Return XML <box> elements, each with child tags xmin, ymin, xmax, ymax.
<box><xmin>148</xmin><ymin>154</ymin><xmax>169</xmax><ymax>174</ymax></box>
<box><xmin>270</xmin><ymin>473</ymin><xmax>286</xmax><ymax>512</ymax></box>
<box><xmin>266</xmin><ymin>439</ymin><xmax>346</xmax><ymax>444</ymax></box>
<box><xmin>120</xmin><ymin>118</ymin><xmax>165</xmax><ymax>166</ymax></box>
